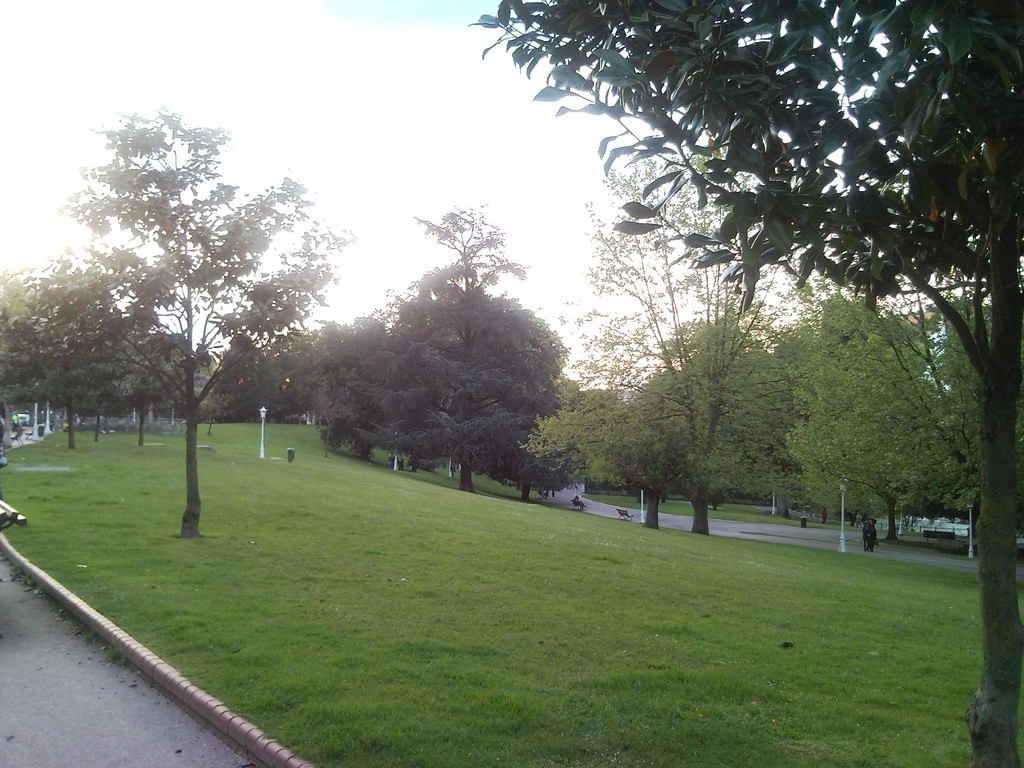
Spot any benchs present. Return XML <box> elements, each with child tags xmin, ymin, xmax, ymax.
<box><xmin>571</xmin><ymin>500</ymin><xmax>586</xmax><ymax>511</ymax></box>
<box><xmin>923</xmin><ymin>530</ymin><xmax>955</xmax><ymax>543</ymax></box>
<box><xmin>616</xmin><ymin>509</ymin><xmax>634</xmax><ymax>520</ymax></box>
<box><xmin>11</xmin><ymin>428</ymin><xmax>25</xmax><ymax>447</ymax></box>
<box><xmin>25</xmin><ymin>432</ymin><xmax>32</xmax><ymax>440</ymax></box>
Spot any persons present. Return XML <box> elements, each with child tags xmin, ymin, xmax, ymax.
<box><xmin>12</xmin><ymin>411</ymin><xmax>19</xmax><ymax>431</ymax></box>
<box><xmin>820</xmin><ymin>506</ymin><xmax>828</xmax><ymax>524</ymax></box>
<box><xmin>538</xmin><ymin>489</ymin><xmax>548</xmax><ymax>499</ymax></box>
<box><xmin>573</xmin><ymin>495</ymin><xmax>587</xmax><ymax>508</ymax></box>
<box><xmin>849</xmin><ymin>511</ymin><xmax>863</xmax><ymax>528</ymax></box>
<box><xmin>862</xmin><ymin>520</ymin><xmax>878</xmax><ymax>551</ymax></box>
<box><xmin>389</xmin><ymin>452</ymin><xmax>404</xmax><ymax>471</ymax></box>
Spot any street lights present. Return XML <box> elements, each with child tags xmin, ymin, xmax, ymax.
<box><xmin>965</xmin><ymin>495</ymin><xmax>974</xmax><ymax>559</ymax></box>
<box><xmin>259</xmin><ymin>406</ymin><xmax>268</xmax><ymax>459</ymax></box>
<box><xmin>839</xmin><ymin>473</ymin><xmax>848</xmax><ymax>553</ymax></box>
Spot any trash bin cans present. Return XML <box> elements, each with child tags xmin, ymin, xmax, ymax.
<box><xmin>800</xmin><ymin>518</ymin><xmax>807</xmax><ymax>528</ymax></box>
<box><xmin>287</xmin><ymin>447</ymin><xmax>295</xmax><ymax>463</ymax></box>
<box><xmin>38</xmin><ymin>424</ymin><xmax>45</xmax><ymax>437</ymax></box>
